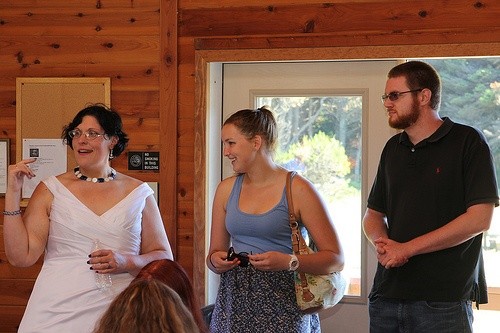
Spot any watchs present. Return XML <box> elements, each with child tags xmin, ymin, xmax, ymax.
<box><xmin>287</xmin><ymin>254</ymin><xmax>300</xmax><ymax>272</ymax></box>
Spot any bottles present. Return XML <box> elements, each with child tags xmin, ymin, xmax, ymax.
<box><xmin>89</xmin><ymin>238</ymin><xmax>113</xmax><ymax>289</ymax></box>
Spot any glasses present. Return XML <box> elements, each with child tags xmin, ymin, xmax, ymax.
<box><xmin>68</xmin><ymin>129</ymin><xmax>107</xmax><ymax>141</ymax></box>
<box><xmin>381</xmin><ymin>89</ymin><xmax>422</xmax><ymax>103</ymax></box>
<box><xmin>226</xmin><ymin>247</ymin><xmax>252</xmax><ymax>267</ymax></box>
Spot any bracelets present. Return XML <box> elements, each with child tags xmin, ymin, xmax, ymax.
<box><xmin>2</xmin><ymin>209</ymin><xmax>22</xmax><ymax>216</ymax></box>
<box><xmin>210</xmin><ymin>252</ymin><xmax>216</xmax><ymax>269</ymax></box>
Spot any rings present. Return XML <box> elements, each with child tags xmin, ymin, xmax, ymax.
<box><xmin>107</xmin><ymin>263</ymin><xmax>111</xmax><ymax>268</ymax></box>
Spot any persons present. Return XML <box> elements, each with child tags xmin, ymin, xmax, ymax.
<box><xmin>91</xmin><ymin>278</ymin><xmax>201</xmax><ymax>333</ymax></box>
<box><xmin>131</xmin><ymin>259</ymin><xmax>212</xmax><ymax>333</ymax></box>
<box><xmin>2</xmin><ymin>102</ymin><xmax>173</xmax><ymax>332</ymax></box>
<box><xmin>362</xmin><ymin>61</ymin><xmax>500</xmax><ymax>333</ymax></box>
<box><xmin>205</xmin><ymin>106</ymin><xmax>344</xmax><ymax>333</ymax></box>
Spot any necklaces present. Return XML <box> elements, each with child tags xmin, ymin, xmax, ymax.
<box><xmin>74</xmin><ymin>165</ymin><xmax>117</xmax><ymax>182</ymax></box>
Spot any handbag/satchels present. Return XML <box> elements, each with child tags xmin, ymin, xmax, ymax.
<box><xmin>286</xmin><ymin>171</ymin><xmax>348</xmax><ymax>314</ymax></box>
